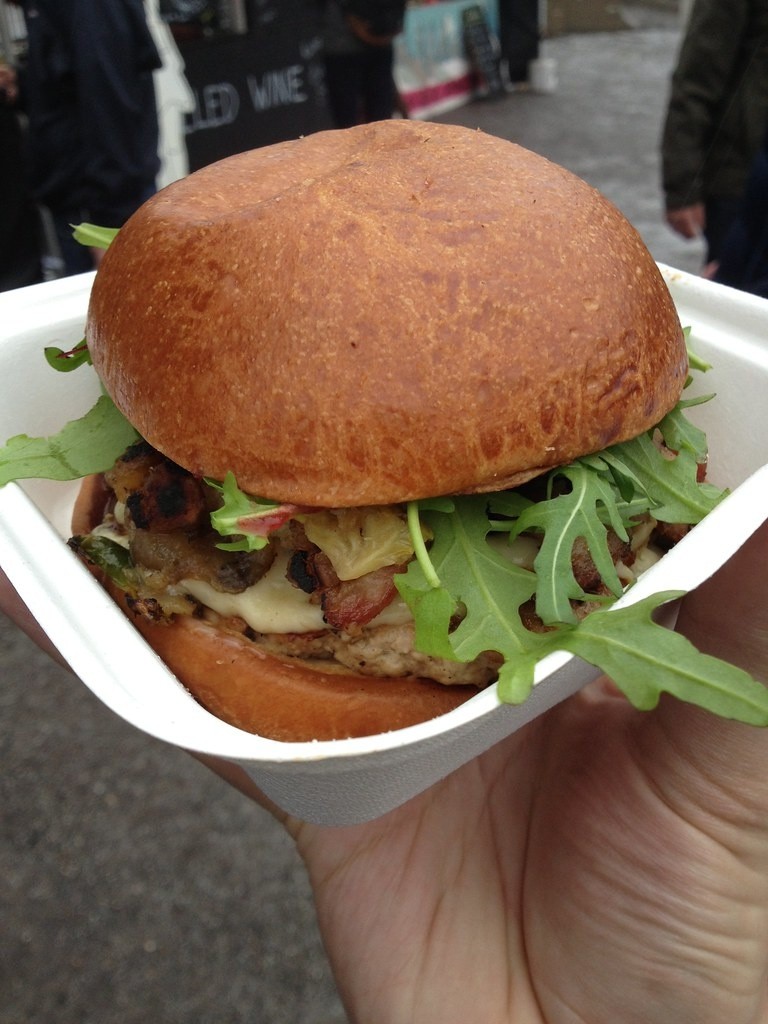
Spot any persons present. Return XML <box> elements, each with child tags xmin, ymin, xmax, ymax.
<box><xmin>0</xmin><ymin>518</ymin><xmax>768</xmax><ymax>1024</ymax></box>
<box><xmin>0</xmin><ymin>0</ymin><xmax>404</xmax><ymax>293</ymax></box>
<box><xmin>496</xmin><ymin>0</ymin><xmax>540</xmax><ymax>93</ymax></box>
<box><xmin>661</xmin><ymin>0</ymin><xmax>768</xmax><ymax>299</ymax></box>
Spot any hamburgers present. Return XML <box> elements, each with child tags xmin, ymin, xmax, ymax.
<box><xmin>0</xmin><ymin>118</ymin><xmax>766</xmax><ymax>739</ymax></box>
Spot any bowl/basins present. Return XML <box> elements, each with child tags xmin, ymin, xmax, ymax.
<box><xmin>0</xmin><ymin>267</ymin><xmax>768</xmax><ymax>824</ymax></box>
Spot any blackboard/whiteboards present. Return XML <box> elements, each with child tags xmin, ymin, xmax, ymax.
<box><xmin>177</xmin><ymin>30</ymin><xmax>336</xmax><ymax>172</ymax></box>
